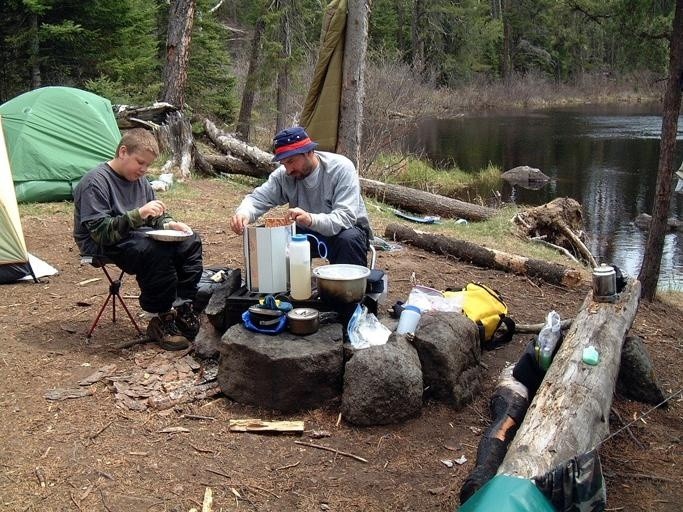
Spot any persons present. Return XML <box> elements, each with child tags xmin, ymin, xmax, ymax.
<box><xmin>230</xmin><ymin>127</ymin><xmax>373</xmax><ymax>267</ymax></box>
<box><xmin>73</xmin><ymin>129</ymin><xmax>201</xmax><ymax>351</ymax></box>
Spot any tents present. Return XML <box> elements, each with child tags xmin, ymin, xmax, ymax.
<box><xmin>0</xmin><ymin>86</ymin><xmax>122</xmax><ymax>203</ymax></box>
<box><xmin>0</xmin><ymin>117</ymin><xmax>39</xmax><ymax>284</ymax></box>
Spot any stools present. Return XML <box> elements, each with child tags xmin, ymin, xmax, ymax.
<box><xmin>79</xmin><ymin>252</ymin><xmax>144</xmax><ymax>339</ymax></box>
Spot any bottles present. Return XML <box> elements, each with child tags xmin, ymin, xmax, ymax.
<box><xmin>544</xmin><ymin>347</ymin><xmax>550</xmax><ymax>369</ymax></box>
<box><xmin>289</xmin><ymin>232</ymin><xmax>311</xmax><ymax>301</ymax></box>
<box><xmin>583</xmin><ymin>346</ymin><xmax>598</xmax><ymax>366</ymax></box>
<box><xmin>592</xmin><ymin>262</ymin><xmax>617</xmax><ymax>301</ymax></box>
<box><xmin>396</xmin><ymin>305</ymin><xmax>421</xmax><ymax>334</ymax></box>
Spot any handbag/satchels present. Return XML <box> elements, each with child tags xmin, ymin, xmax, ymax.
<box><xmin>242</xmin><ymin>295</ymin><xmax>293</xmax><ymax>336</ymax></box>
<box><xmin>440</xmin><ymin>281</ymin><xmax>514</xmax><ymax>351</ymax></box>
<box><xmin>513</xmin><ymin>330</ymin><xmax>565</xmax><ymax>396</ymax></box>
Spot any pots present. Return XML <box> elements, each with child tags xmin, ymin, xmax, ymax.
<box><xmin>313</xmin><ymin>263</ymin><xmax>371</xmax><ymax>304</ymax></box>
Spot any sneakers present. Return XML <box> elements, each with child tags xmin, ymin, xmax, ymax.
<box><xmin>173</xmin><ymin>301</ymin><xmax>199</xmax><ymax>338</ymax></box>
<box><xmin>146</xmin><ymin>311</ymin><xmax>189</xmax><ymax>351</ymax></box>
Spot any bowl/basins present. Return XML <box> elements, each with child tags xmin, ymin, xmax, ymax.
<box><xmin>287</xmin><ymin>308</ymin><xmax>319</xmax><ymax>335</ymax></box>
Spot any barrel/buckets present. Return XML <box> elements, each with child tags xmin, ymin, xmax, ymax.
<box><xmin>592</xmin><ymin>266</ymin><xmax>618</xmax><ymax>303</ymax></box>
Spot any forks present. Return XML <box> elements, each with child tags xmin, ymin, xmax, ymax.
<box><xmin>162</xmin><ymin>211</ymin><xmax>170</xmax><ymax>230</ymax></box>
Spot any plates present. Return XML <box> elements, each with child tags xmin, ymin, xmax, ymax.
<box><xmin>146</xmin><ymin>230</ymin><xmax>193</xmax><ymax>242</ymax></box>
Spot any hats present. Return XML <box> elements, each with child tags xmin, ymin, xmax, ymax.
<box><xmin>272</xmin><ymin>126</ymin><xmax>319</xmax><ymax>162</ymax></box>
